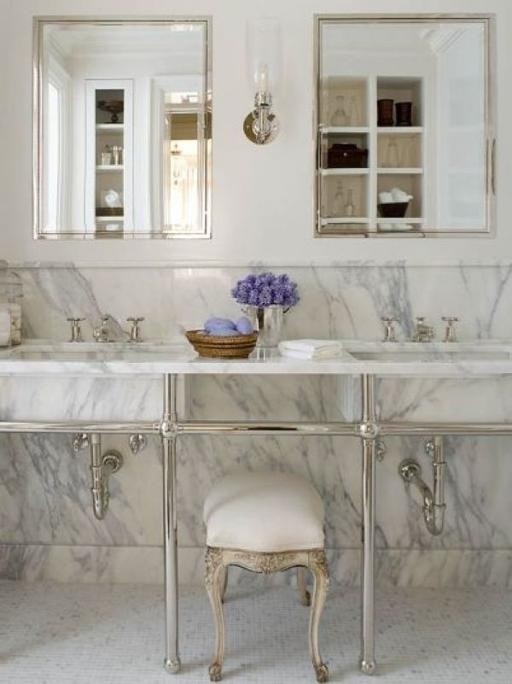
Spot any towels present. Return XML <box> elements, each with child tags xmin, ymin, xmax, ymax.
<box><xmin>278</xmin><ymin>338</ymin><xmax>343</xmax><ymax>360</ymax></box>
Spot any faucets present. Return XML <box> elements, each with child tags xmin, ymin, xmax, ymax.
<box><xmin>412</xmin><ymin>313</ymin><xmax>434</xmax><ymax>342</ymax></box>
<box><xmin>93</xmin><ymin>313</ymin><xmax>116</xmax><ymax>344</ymax></box>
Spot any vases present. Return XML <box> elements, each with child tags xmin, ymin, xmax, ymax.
<box><xmin>386</xmin><ymin>134</ymin><xmax>413</xmax><ymax>168</ymax></box>
<box><xmin>330</xmin><ymin>180</ymin><xmax>356</xmax><ymax>218</ymax></box>
<box><xmin>330</xmin><ymin>96</ymin><xmax>360</xmax><ymax>126</ymax></box>
<box><xmin>255</xmin><ymin>304</ymin><xmax>283</xmax><ymax>347</ymax></box>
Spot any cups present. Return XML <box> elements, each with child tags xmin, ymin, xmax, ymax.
<box><xmin>394</xmin><ymin>101</ymin><xmax>413</xmax><ymax>127</ymax></box>
<box><xmin>376</xmin><ymin>98</ymin><xmax>395</xmax><ymax>127</ymax></box>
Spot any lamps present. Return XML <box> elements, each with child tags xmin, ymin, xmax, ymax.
<box><xmin>243</xmin><ymin>68</ymin><xmax>281</xmax><ymax>146</ymax></box>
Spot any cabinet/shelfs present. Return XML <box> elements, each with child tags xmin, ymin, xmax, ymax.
<box><xmin>86</xmin><ymin>79</ymin><xmax>133</xmax><ymax>232</ymax></box>
<box><xmin>324</xmin><ymin>73</ymin><xmax>428</xmax><ymax>230</ymax></box>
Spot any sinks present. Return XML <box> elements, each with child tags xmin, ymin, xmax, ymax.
<box><xmin>6</xmin><ymin>347</ymin><xmax>176</xmax><ymax>366</ymax></box>
<box><xmin>341</xmin><ymin>346</ymin><xmax>512</xmax><ymax>365</ymax></box>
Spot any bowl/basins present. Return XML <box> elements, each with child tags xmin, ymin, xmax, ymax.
<box><xmin>378</xmin><ymin>202</ymin><xmax>410</xmax><ymax>218</ymax></box>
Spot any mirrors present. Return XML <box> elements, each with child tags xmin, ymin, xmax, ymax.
<box><xmin>314</xmin><ymin>11</ymin><xmax>495</xmax><ymax>238</ymax></box>
<box><xmin>32</xmin><ymin>14</ymin><xmax>213</xmax><ymax>240</ymax></box>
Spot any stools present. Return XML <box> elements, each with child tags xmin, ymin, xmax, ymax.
<box><xmin>202</xmin><ymin>471</ymin><xmax>330</xmax><ymax>683</ymax></box>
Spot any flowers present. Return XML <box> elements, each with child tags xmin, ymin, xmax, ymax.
<box><xmin>230</xmin><ymin>271</ymin><xmax>301</xmax><ymax>329</ymax></box>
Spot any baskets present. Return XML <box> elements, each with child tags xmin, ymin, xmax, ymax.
<box><xmin>185</xmin><ymin>330</ymin><xmax>257</xmax><ymax>357</ymax></box>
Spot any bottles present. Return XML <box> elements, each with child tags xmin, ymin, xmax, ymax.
<box><xmin>386</xmin><ymin>135</ymin><xmax>401</xmax><ymax>169</ymax></box>
<box><xmin>333</xmin><ymin>179</ymin><xmax>354</xmax><ymax>218</ymax></box>
<box><xmin>331</xmin><ymin>93</ymin><xmax>359</xmax><ymax>127</ymax></box>
